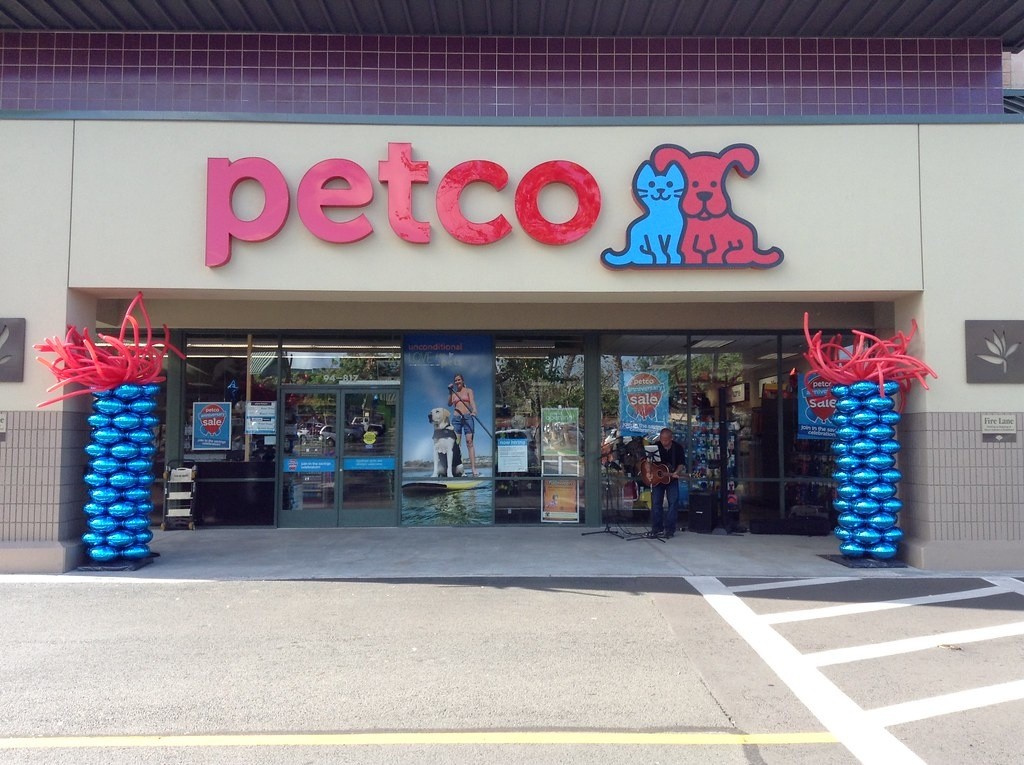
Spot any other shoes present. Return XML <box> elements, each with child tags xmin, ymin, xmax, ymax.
<box><xmin>646</xmin><ymin>529</ymin><xmax>664</xmax><ymax>536</ymax></box>
<box><xmin>664</xmin><ymin>531</ymin><xmax>675</xmax><ymax>538</ymax></box>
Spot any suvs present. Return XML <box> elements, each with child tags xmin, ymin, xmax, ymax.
<box><xmin>183</xmin><ymin>415</ymin><xmax>384</xmax><ymax>455</ymax></box>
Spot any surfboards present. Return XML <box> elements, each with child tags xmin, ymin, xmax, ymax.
<box><xmin>402</xmin><ymin>475</ymin><xmax>484</xmax><ymax>490</ymax></box>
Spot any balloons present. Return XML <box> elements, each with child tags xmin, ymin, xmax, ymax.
<box><xmin>800</xmin><ymin>312</ymin><xmax>938</xmax><ymax>560</ymax></box>
<box><xmin>33</xmin><ymin>291</ymin><xmax>186</xmax><ymax>561</ymax></box>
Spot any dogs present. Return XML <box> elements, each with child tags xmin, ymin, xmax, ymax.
<box><xmin>428</xmin><ymin>408</ymin><xmax>464</xmax><ymax>478</ymax></box>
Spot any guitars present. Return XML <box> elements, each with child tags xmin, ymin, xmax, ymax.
<box><xmin>640</xmin><ymin>460</ymin><xmax>702</xmax><ymax>488</ymax></box>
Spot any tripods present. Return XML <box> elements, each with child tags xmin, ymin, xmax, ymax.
<box><xmin>581</xmin><ymin>439</ymin><xmax>666</xmax><ymax>543</ymax></box>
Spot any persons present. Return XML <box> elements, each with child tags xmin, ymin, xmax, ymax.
<box><xmin>643</xmin><ymin>428</ymin><xmax>685</xmax><ymax>539</ymax></box>
<box><xmin>448</xmin><ymin>374</ymin><xmax>479</xmax><ymax>477</ymax></box>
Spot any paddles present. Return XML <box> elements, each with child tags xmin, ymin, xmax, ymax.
<box><xmin>448</xmin><ymin>386</ymin><xmax>492</xmax><ymax>439</ymax></box>
